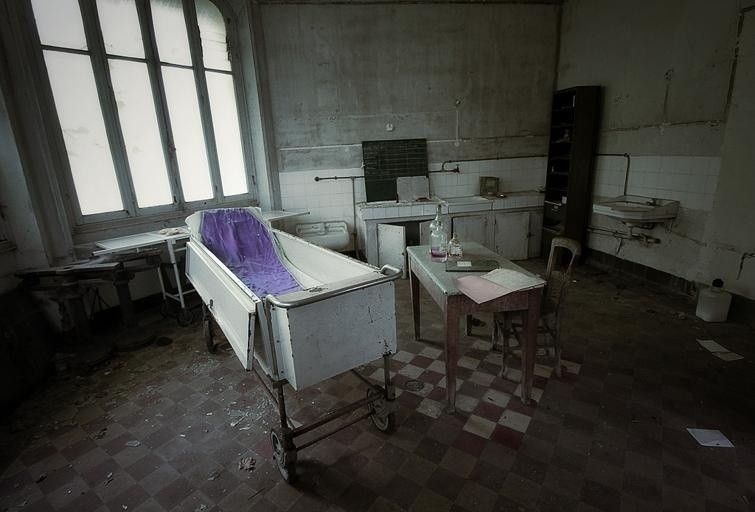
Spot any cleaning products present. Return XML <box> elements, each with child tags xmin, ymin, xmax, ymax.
<box><xmin>429</xmin><ymin>196</ymin><xmax>450</xmax><ymax>262</ymax></box>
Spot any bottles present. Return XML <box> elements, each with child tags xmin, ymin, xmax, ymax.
<box><xmin>448</xmin><ymin>232</ymin><xmax>463</xmax><ymax>258</ymax></box>
<box><xmin>695</xmin><ymin>278</ymin><xmax>732</xmax><ymax>322</ymax></box>
<box><xmin>429</xmin><ymin>203</ymin><xmax>448</xmax><ymax>263</ymax></box>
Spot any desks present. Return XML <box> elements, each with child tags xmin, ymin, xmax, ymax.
<box><xmin>407</xmin><ymin>242</ymin><xmax>546</xmax><ymax>412</ymax></box>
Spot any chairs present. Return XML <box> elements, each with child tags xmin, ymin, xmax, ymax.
<box><xmin>492</xmin><ymin>237</ymin><xmax>581</xmax><ymax>379</ymax></box>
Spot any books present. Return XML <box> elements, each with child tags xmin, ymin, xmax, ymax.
<box><xmin>445</xmin><ymin>260</ymin><xmax>500</xmax><ymax>272</ymax></box>
<box><xmin>451</xmin><ymin>266</ymin><xmax>548</xmax><ymax>305</ymax></box>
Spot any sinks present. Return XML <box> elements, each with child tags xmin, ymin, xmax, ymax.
<box><xmin>593</xmin><ymin>195</ymin><xmax>681</xmax><ymax>225</ymax></box>
<box><xmin>295</xmin><ymin>222</ymin><xmax>351</xmax><ymax>250</ymax></box>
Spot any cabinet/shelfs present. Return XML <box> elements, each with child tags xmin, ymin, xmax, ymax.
<box><xmin>355</xmin><ymin>210</ymin><xmax>541</xmax><ymax>281</ymax></box>
<box><xmin>543</xmin><ymin>85</ymin><xmax>605</xmax><ymax>268</ymax></box>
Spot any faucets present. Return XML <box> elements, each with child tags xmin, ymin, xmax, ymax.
<box><xmin>646</xmin><ymin>198</ymin><xmax>657</xmax><ymax>206</ymax></box>
